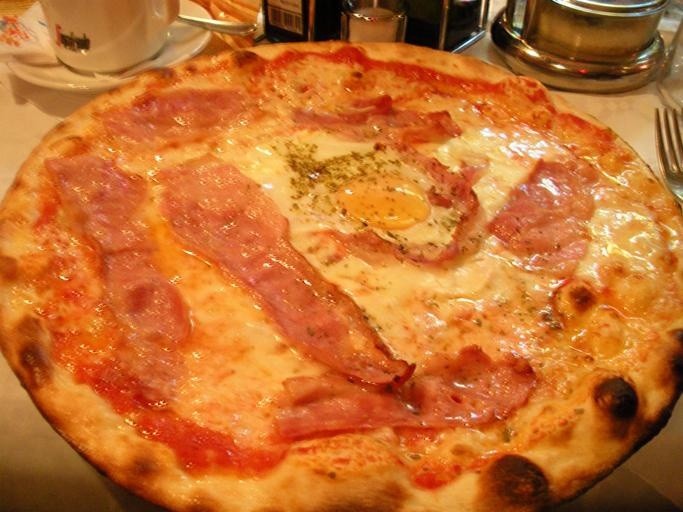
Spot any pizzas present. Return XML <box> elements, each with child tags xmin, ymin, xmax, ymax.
<box><xmin>0</xmin><ymin>39</ymin><xmax>683</xmax><ymax>512</ymax></box>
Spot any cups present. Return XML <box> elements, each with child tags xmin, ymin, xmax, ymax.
<box><xmin>39</xmin><ymin>0</ymin><xmax>180</xmax><ymax>72</ymax></box>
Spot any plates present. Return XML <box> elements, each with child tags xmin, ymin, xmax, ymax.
<box><xmin>5</xmin><ymin>1</ymin><xmax>214</xmax><ymax>90</ymax></box>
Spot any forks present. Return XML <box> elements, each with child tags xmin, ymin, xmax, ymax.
<box><xmin>654</xmin><ymin>107</ymin><xmax>683</xmax><ymax>203</ymax></box>
